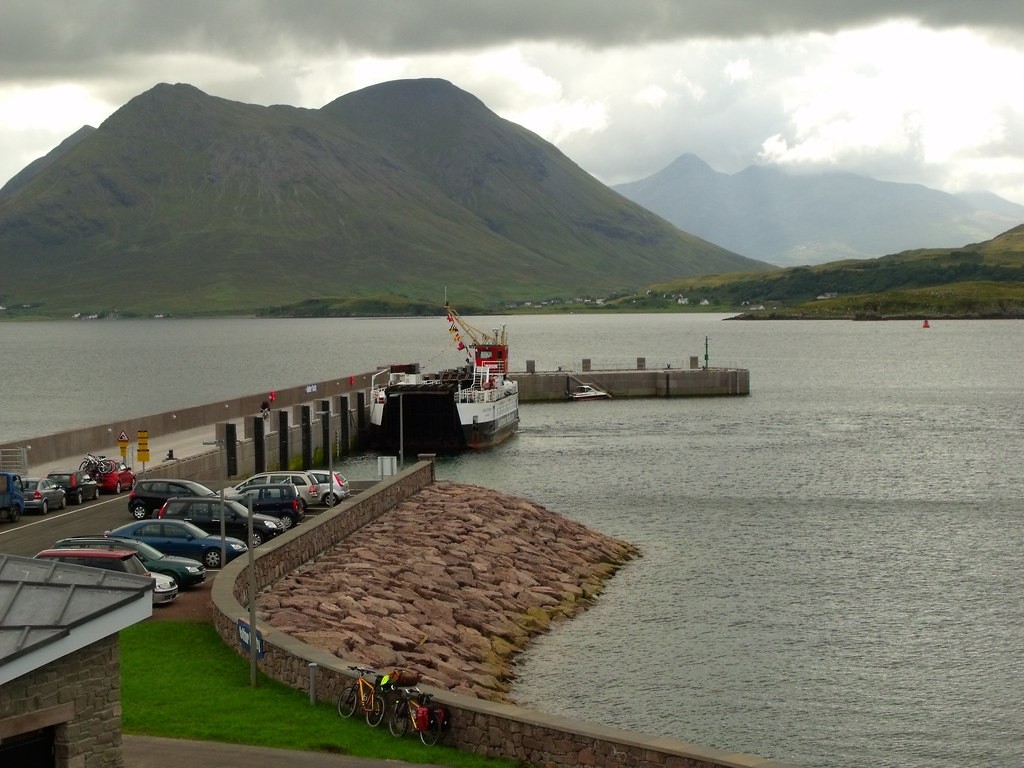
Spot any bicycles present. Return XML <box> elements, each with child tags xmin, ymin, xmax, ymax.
<box><xmin>337</xmin><ymin>666</ymin><xmax>386</xmax><ymax>727</ymax></box>
<box><xmin>79</xmin><ymin>453</ymin><xmax>116</xmax><ymax>474</ymax></box>
<box><xmin>389</xmin><ymin>684</ymin><xmax>441</xmax><ymax>748</ymax></box>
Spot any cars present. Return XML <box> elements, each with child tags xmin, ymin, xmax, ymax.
<box><xmin>46</xmin><ymin>469</ymin><xmax>99</xmax><ymax>505</ymax></box>
<box><xmin>276</xmin><ymin>469</ymin><xmax>351</xmax><ymax>508</ymax></box>
<box><xmin>102</xmin><ymin>519</ymin><xmax>249</xmax><ymax>569</ymax></box>
<box><xmin>85</xmin><ymin>460</ymin><xmax>137</xmax><ymax>494</ymax></box>
<box><xmin>14</xmin><ymin>475</ymin><xmax>67</xmax><ymax>515</ymax></box>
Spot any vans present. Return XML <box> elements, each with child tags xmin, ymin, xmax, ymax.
<box><xmin>32</xmin><ymin>549</ymin><xmax>178</xmax><ymax>607</ymax></box>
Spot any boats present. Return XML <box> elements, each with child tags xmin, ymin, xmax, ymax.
<box><xmin>569</xmin><ymin>384</ymin><xmax>608</xmax><ymax>400</ymax></box>
<box><xmin>367</xmin><ymin>300</ymin><xmax>520</xmax><ymax>450</ymax></box>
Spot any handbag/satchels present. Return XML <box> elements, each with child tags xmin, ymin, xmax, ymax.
<box><xmin>416</xmin><ymin>706</ymin><xmax>434</xmax><ymax>731</ymax></box>
<box><xmin>389</xmin><ymin>668</ymin><xmax>422</xmax><ymax>686</ymax></box>
<box><xmin>433</xmin><ymin>707</ymin><xmax>449</xmax><ymax>732</ymax></box>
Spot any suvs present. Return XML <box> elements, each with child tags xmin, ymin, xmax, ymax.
<box><xmin>234</xmin><ymin>483</ymin><xmax>304</xmax><ymax>529</ymax></box>
<box><xmin>215</xmin><ymin>470</ymin><xmax>323</xmax><ymax>506</ymax></box>
<box><xmin>48</xmin><ymin>535</ymin><xmax>207</xmax><ymax>592</ymax></box>
<box><xmin>128</xmin><ymin>479</ymin><xmax>233</xmax><ymax>521</ymax></box>
<box><xmin>150</xmin><ymin>496</ymin><xmax>287</xmax><ymax>548</ymax></box>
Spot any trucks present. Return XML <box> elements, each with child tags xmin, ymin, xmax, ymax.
<box><xmin>0</xmin><ymin>471</ymin><xmax>26</xmax><ymax>524</ymax></box>
